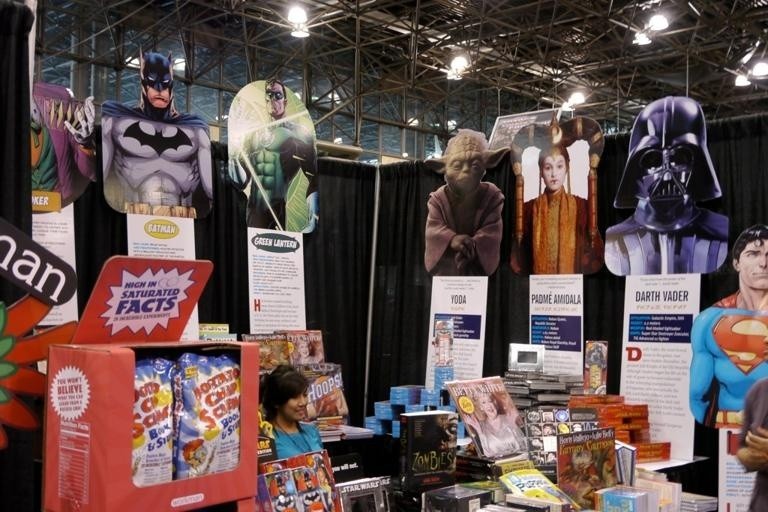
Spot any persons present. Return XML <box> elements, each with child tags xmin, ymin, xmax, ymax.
<box><xmin>226</xmin><ymin>78</ymin><xmax>320</xmax><ymax>236</ymax></box>
<box><xmin>100</xmin><ymin>44</ymin><xmax>214</xmax><ymax>219</ymax></box>
<box><xmin>736</xmin><ymin>376</ymin><xmax>768</xmax><ymax>511</ymax></box>
<box><xmin>259</xmin><ymin>366</ymin><xmax>324</xmax><ymax>460</ymax></box>
<box><xmin>510</xmin><ymin>117</ymin><xmax>607</xmax><ymax>278</ymax></box>
<box><xmin>296</xmin><ymin>337</ymin><xmax>320</xmax><ymax>368</ymax></box>
<box><xmin>463</xmin><ymin>389</ymin><xmax>526</xmax><ymax>457</ymax></box>
<box><xmin>689</xmin><ymin>224</ymin><xmax>768</xmax><ymax>427</ymax></box>
<box><xmin>31</xmin><ymin>81</ymin><xmax>97</xmax><ymax>213</ymax></box>
<box><xmin>429</xmin><ymin>414</ymin><xmax>458</xmax><ymax>471</ymax></box>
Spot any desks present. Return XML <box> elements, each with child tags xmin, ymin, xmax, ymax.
<box><xmin>322</xmin><ymin>433</ymin><xmax>722</xmax><ymax>512</ymax></box>
